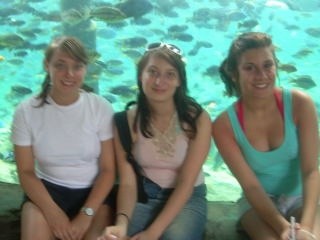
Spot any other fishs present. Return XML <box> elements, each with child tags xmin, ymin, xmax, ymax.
<box><xmin>0</xmin><ymin>0</ymin><xmax>320</xmax><ymax>194</ymax></box>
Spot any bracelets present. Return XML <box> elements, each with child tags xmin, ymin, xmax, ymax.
<box><xmin>113</xmin><ymin>212</ymin><xmax>132</xmax><ymax>226</ymax></box>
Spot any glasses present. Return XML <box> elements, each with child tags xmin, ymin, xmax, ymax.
<box><xmin>145</xmin><ymin>42</ymin><xmax>180</xmax><ymax>55</ymax></box>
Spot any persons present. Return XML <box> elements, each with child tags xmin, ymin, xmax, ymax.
<box><xmin>101</xmin><ymin>42</ymin><xmax>212</xmax><ymax>240</ymax></box>
<box><xmin>10</xmin><ymin>37</ymin><xmax>116</xmax><ymax>240</ymax></box>
<box><xmin>213</xmin><ymin>33</ymin><xmax>320</xmax><ymax>240</ymax></box>
<box><xmin>79</xmin><ymin>207</ymin><xmax>94</xmax><ymax>218</ymax></box>
